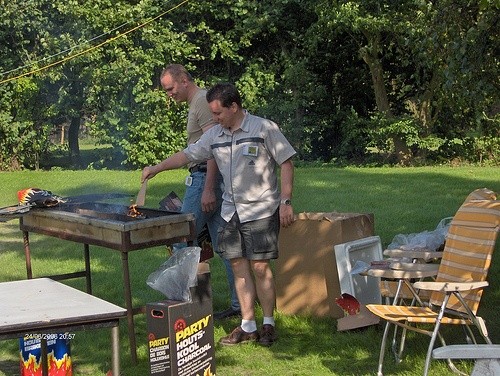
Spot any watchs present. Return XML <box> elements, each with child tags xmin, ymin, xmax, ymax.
<box><xmin>280</xmin><ymin>199</ymin><xmax>291</xmax><ymax>206</ymax></box>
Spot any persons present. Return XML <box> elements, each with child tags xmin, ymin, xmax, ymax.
<box><xmin>140</xmin><ymin>83</ymin><xmax>297</xmax><ymax>346</ymax></box>
<box><xmin>160</xmin><ymin>64</ymin><xmax>242</xmax><ymax>320</ymax></box>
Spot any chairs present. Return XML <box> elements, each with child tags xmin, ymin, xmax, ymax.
<box><xmin>359</xmin><ymin>188</ymin><xmax>500</xmax><ymax>376</ymax></box>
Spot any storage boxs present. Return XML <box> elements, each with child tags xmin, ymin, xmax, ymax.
<box><xmin>274</xmin><ymin>211</ymin><xmax>374</xmax><ymax>320</ymax></box>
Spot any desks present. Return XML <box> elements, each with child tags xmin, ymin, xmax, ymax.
<box><xmin>0</xmin><ymin>278</ymin><xmax>128</xmax><ymax>376</ymax></box>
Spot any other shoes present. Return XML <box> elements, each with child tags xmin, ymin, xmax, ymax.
<box><xmin>213</xmin><ymin>307</ymin><xmax>241</xmax><ymax>318</ymax></box>
<box><xmin>218</xmin><ymin>326</ymin><xmax>259</xmax><ymax>344</ymax></box>
<box><xmin>260</xmin><ymin>324</ymin><xmax>277</xmax><ymax>346</ymax></box>
